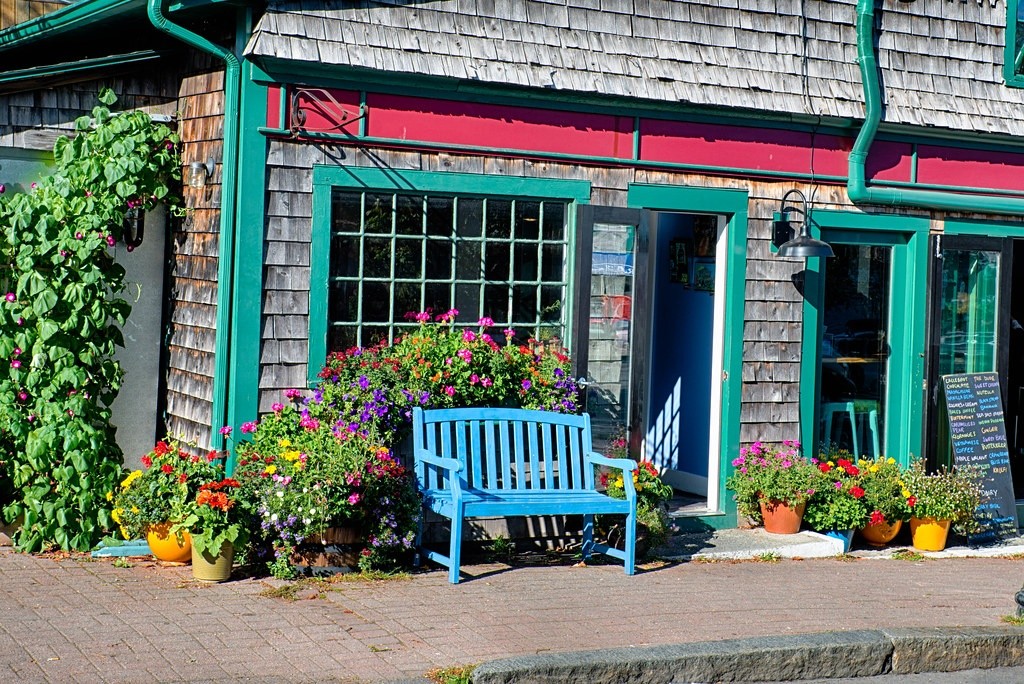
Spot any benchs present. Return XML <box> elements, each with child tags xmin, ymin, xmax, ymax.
<box><xmin>410</xmin><ymin>406</ymin><xmax>639</xmax><ymax>577</ymax></box>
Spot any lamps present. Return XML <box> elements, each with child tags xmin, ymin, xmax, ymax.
<box><xmin>769</xmin><ymin>188</ymin><xmax>838</xmax><ymax>258</ymax></box>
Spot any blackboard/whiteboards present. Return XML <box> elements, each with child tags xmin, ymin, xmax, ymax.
<box><xmin>942</xmin><ymin>371</ymin><xmax>1019</xmax><ymax>548</ymax></box>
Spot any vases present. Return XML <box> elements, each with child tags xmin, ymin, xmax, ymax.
<box><xmin>757</xmin><ymin>490</ymin><xmax>806</xmax><ymax>533</ymax></box>
<box><xmin>272</xmin><ymin>526</ymin><xmax>372</xmax><ymax>568</ymax></box>
<box><xmin>860</xmin><ymin>510</ymin><xmax>903</xmax><ymax>547</ymax></box>
<box><xmin>137</xmin><ymin>518</ymin><xmax>193</xmax><ymax>567</ymax></box>
<box><xmin>824</xmin><ymin>521</ymin><xmax>856</xmax><ymax>553</ymax></box>
<box><xmin>188</xmin><ymin>532</ymin><xmax>234</xmax><ymax>580</ymax></box>
<box><xmin>908</xmin><ymin>514</ymin><xmax>953</xmax><ymax>550</ymax></box>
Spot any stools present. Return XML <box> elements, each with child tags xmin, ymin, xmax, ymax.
<box><xmin>823</xmin><ymin>402</ymin><xmax>879</xmax><ymax>463</ymax></box>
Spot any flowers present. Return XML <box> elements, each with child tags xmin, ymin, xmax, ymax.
<box><xmin>727</xmin><ymin>437</ymin><xmax>990</xmax><ymax>524</ymax></box>
<box><xmin>107</xmin><ymin>310</ymin><xmax>675</xmax><ymax>577</ymax></box>
